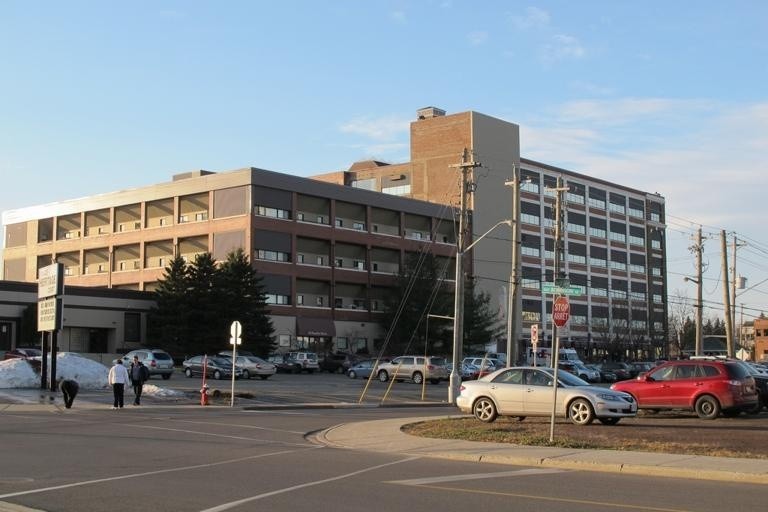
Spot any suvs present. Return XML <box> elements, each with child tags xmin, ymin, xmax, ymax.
<box><xmin>112</xmin><ymin>349</ymin><xmax>177</xmax><ymax>380</ymax></box>
<box><xmin>375</xmin><ymin>355</ymin><xmax>448</xmax><ymax>384</ymax></box>
<box><xmin>217</xmin><ymin>349</ymin><xmax>253</xmax><ymax>358</ymax></box>
<box><xmin>318</xmin><ymin>354</ymin><xmax>353</xmax><ymax>373</ymax></box>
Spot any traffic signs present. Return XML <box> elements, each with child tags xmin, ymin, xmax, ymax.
<box><xmin>539</xmin><ymin>286</ymin><xmax>583</xmax><ymax>296</ymax></box>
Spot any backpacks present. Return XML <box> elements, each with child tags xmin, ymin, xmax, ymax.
<box><xmin>141</xmin><ymin>364</ymin><xmax>150</xmax><ymax>381</ymax></box>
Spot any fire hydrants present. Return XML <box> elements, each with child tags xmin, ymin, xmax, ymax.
<box><xmin>199</xmin><ymin>383</ymin><xmax>211</xmax><ymax>406</ymax></box>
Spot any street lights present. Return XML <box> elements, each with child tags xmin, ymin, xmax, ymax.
<box><xmin>442</xmin><ymin>149</ymin><xmax>517</xmax><ymax>409</ymax></box>
<box><xmin>680</xmin><ymin>227</ymin><xmax>705</xmax><ymax>357</ymax></box>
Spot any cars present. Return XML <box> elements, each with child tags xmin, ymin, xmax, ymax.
<box><xmin>266</xmin><ymin>355</ymin><xmax>301</xmax><ymax>374</ymax></box>
<box><xmin>4</xmin><ymin>347</ymin><xmax>42</xmax><ymax>370</ymax></box>
<box><xmin>225</xmin><ymin>355</ymin><xmax>277</xmax><ymax>380</ymax></box>
<box><xmin>447</xmin><ymin>362</ymin><xmax>479</xmax><ymax>380</ymax></box>
<box><xmin>347</xmin><ymin>359</ymin><xmax>387</xmax><ymax>378</ymax></box>
<box><xmin>472</xmin><ymin>365</ymin><xmax>504</xmax><ymax>380</ymax></box>
<box><xmin>181</xmin><ymin>356</ymin><xmax>244</xmax><ymax>381</ymax></box>
<box><xmin>454</xmin><ymin>367</ymin><xmax>639</xmax><ymax>426</ymax></box>
<box><xmin>574</xmin><ymin>359</ymin><xmax>768</xmax><ymax>420</ymax></box>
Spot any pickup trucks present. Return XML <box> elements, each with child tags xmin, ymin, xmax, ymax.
<box><xmin>286</xmin><ymin>352</ymin><xmax>320</xmax><ymax>373</ymax></box>
<box><xmin>463</xmin><ymin>357</ymin><xmax>504</xmax><ymax>369</ymax></box>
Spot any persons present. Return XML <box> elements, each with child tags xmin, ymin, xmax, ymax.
<box><xmin>129</xmin><ymin>356</ymin><xmax>146</xmax><ymax>406</ymax></box>
<box><xmin>107</xmin><ymin>359</ymin><xmax>130</xmax><ymax>409</ymax></box>
<box><xmin>56</xmin><ymin>374</ymin><xmax>79</xmax><ymax>409</ymax></box>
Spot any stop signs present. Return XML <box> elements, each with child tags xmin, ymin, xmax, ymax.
<box><xmin>552</xmin><ymin>297</ymin><xmax>570</xmax><ymax>328</ymax></box>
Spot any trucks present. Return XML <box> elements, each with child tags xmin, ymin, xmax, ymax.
<box><xmin>526</xmin><ymin>347</ymin><xmax>584</xmax><ymax>367</ymax></box>
